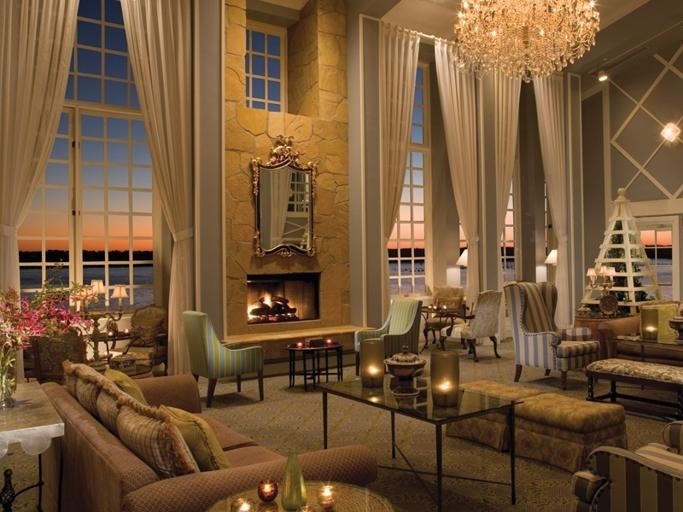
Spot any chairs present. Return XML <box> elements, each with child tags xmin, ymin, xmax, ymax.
<box><xmin>32</xmin><ymin>327</ymin><xmax>87</xmax><ymax>380</ymax></box>
<box><xmin>439</xmin><ymin>290</ymin><xmax>503</xmax><ymax>361</ymax></box>
<box><xmin>110</xmin><ymin>306</ymin><xmax>169</xmax><ymax>374</ymax></box>
<box><xmin>420</xmin><ymin>287</ymin><xmax>467</xmax><ymax>353</ymax></box>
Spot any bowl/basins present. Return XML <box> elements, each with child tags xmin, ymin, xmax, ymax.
<box><xmin>381</xmin><ymin>346</ymin><xmax>426</xmax><ymax>379</ymax></box>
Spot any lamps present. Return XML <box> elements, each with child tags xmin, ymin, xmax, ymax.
<box><xmin>543</xmin><ymin>250</ymin><xmax>557</xmax><ymax>266</ymax></box>
<box><xmin>456</xmin><ymin>249</ymin><xmax>467</xmax><ymax>266</ymax></box>
<box><xmin>451</xmin><ymin>2</ymin><xmax>600</xmax><ymax>82</ymax></box>
<box><xmin>586</xmin><ymin>265</ymin><xmax>617</xmax><ymax>300</ymax></box>
<box><xmin>79</xmin><ymin>279</ymin><xmax>130</xmax><ymax>367</ymax></box>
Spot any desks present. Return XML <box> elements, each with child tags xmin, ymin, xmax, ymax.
<box><xmin>287</xmin><ymin>342</ymin><xmax>344</xmax><ymax>391</ymax></box>
<box><xmin>318</xmin><ymin>376</ymin><xmax>523</xmax><ymax>512</ymax></box>
<box><xmin>206</xmin><ymin>480</ymin><xmax>393</xmax><ymax>512</ymax></box>
<box><xmin>574</xmin><ymin>312</ymin><xmax>646</xmax><ymax>359</ymax></box>
<box><xmin>94</xmin><ymin>362</ymin><xmax>152</xmax><ymax>377</ymax></box>
<box><xmin>420</xmin><ymin>307</ymin><xmax>460</xmax><ymax>347</ymax></box>
<box><xmin>83</xmin><ymin>331</ymin><xmax>145</xmax><ymax>361</ymax></box>
<box><xmin>1</xmin><ymin>381</ymin><xmax>64</xmax><ymax>512</ymax></box>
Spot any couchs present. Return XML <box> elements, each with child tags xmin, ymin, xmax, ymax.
<box><xmin>512</xmin><ymin>394</ymin><xmax>627</xmax><ymax>474</ymax></box>
<box><xmin>599</xmin><ymin>301</ymin><xmax>681</xmax><ymax>367</ymax></box>
<box><xmin>503</xmin><ymin>281</ymin><xmax>599</xmax><ymax>390</ymax></box>
<box><xmin>40</xmin><ymin>364</ymin><xmax>377</xmax><ymax>512</ymax></box>
<box><xmin>569</xmin><ymin>421</ymin><xmax>682</xmax><ymax>512</ymax></box>
<box><xmin>353</xmin><ymin>298</ymin><xmax>422</xmax><ymax>373</ymax></box>
<box><xmin>443</xmin><ymin>381</ymin><xmax>543</xmax><ymax>450</ymax></box>
<box><xmin>183</xmin><ymin>310</ymin><xmax>264</xmax><ymax>407</ymax></box>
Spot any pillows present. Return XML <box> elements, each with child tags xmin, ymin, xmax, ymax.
<box><xmin>104</xmin><ymin>368</ymin><xmax>149</xmax><ymax>407</ymax></box>
<box><xmin>97</xmin><ymin>384</ymin><xmax>131</xmax><ymax>431</ymax></box>
<box><xmin>77</xmin><ymin>368</ymin><xmax>106</xmax><ymax>417</ymax></box>
<box><xmin>159</xmin><ymin>405</ymin><xmax>232</xmax><ymax>474</ymax></box>
<box><xmin>117</xmin><ymin>398</ymin><xmax>196</xmax><ymax>477</ymax></box>
<box><xmin>63</xmin><ymin>359</ymin><xmax>89</xmax><ymax>399</ymax></box>
<box><xmin>640</xmin><ymin>303</ymin><xmax>681</xmax><ymax>346</ymax></box>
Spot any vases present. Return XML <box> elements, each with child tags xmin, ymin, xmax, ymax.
<box><xmin>0</xmin><ymin>363</ymin><xmax>17</xmax><ymax>407</ymax></box>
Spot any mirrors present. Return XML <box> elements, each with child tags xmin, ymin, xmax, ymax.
<box><xmin>249</xmin><ymin>135</ymin><xmax>316</xmax><ymax>260</ymax></box>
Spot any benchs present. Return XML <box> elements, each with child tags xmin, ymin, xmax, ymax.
<box><xmin>583</xmin><ymin>357</ymin><xmax>683</xmax><ymax>419</ymax></box>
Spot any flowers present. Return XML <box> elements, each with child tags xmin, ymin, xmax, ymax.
<box><xmin>0</xmin><ymin>262</ymin><xmax>92</xmax><ymax>406</ymax></box>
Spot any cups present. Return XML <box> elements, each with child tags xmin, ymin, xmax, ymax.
<box><xmin>358</xmin><ymin>339</ymin><xmax>385</xmax><ymax>387</ymax></box>
<box><xmin>228</xmin><ymin>478</ymin><xmax>337</xmax><ymax>511</ymax></box>
<box><xmin>638</xmin><ymin>307</ymin><xmax>659</xmax><ymax>339</ymax></box>
<box><xmin>430</xmin><ymin>349</ymin><xmax>461</xmax><ymax>408</ymax></box>
